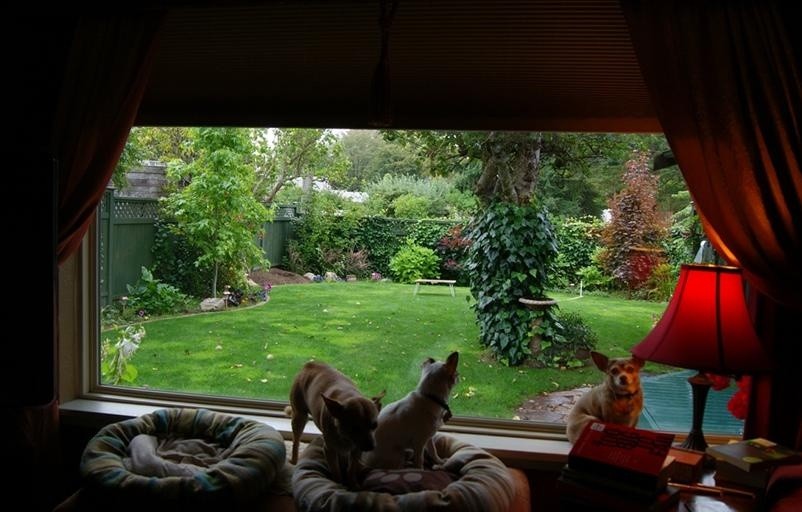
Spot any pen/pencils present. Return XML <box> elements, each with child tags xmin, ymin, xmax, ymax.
<box><xmin>667</xmin><ymin>482</ymin><xmax>755</xmax><ymax>499</ymax></box>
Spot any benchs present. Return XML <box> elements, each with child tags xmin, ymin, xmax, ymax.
<box><xmin>411</xmin><ymin>279</ymin><xmax>456</xmax><ymax>298</ymax></box>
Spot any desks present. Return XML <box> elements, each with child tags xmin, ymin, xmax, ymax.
<box><xmin>531</xmin><ymin>441</ymin><xmax>802</xmax><ymax>512</ymax></box>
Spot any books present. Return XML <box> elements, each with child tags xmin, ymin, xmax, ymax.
<box><xmin>557</xmin><ymin>417</ymin><xmax>802</xmax><ymax>511</ymax></box>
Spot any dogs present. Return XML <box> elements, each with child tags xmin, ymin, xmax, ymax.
<box><xmin>564</xmin><ymin>350</ymin><xmax>645</xmax><ymax>447</ymax></box>
<box><xmin>282</xmin><ymin>358</ymin><xmax>386</xmax><ymax>471</ymax></box>
<box><xmin>375</xmin><ymin>349</ymin><xmax>462</xmax><ymax>470</ymax></box>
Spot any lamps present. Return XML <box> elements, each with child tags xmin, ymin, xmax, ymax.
<box><xmin>626</xmin><ymin>264</ymin><xmax>766</xmax><ymax>474</ymax></box>
